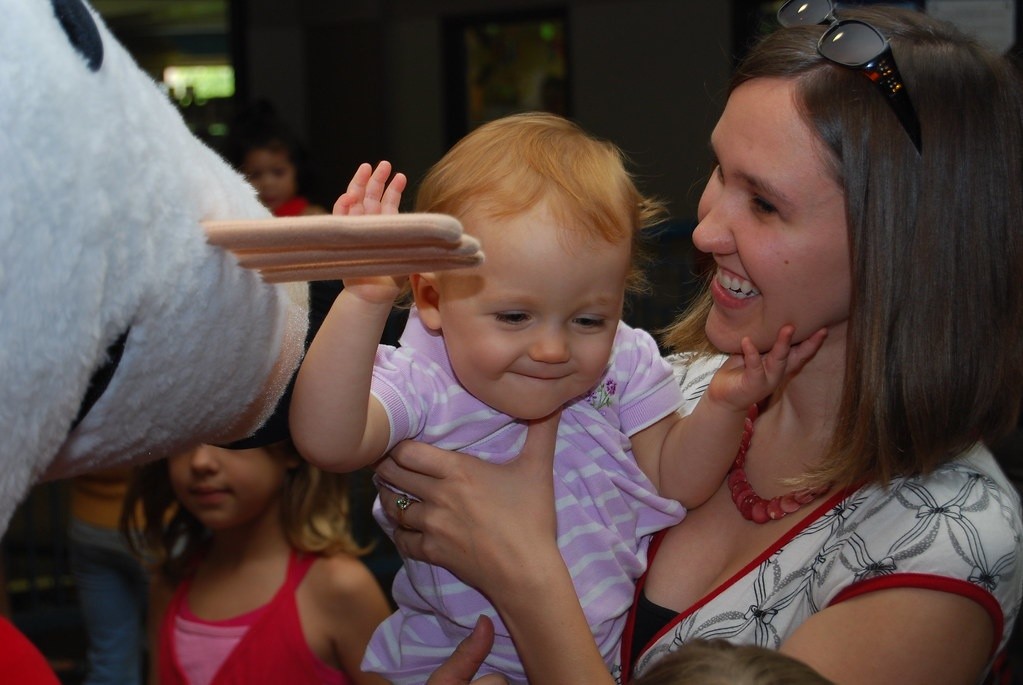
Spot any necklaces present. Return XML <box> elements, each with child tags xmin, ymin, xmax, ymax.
<box><xmin>726</xmin><ymin>398</ymin><xmax>841</xmax><ymax>523</ymax></box>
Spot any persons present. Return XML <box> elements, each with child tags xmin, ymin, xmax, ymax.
<box><xmin>122</xmin><ymin>425</ymin><xmax>393</xmax><ymax>685</ymax></box>
<box><xmin>370</xmin><ymin>0</ymin><xmax>1023</xmax><ymax>685</ymax></box>
<box><xmin>629</xmin><ymin>636</ymin><xmax>838</xmax><ymax>685</ymax></box>
<box><xmin>237</xmin><ymin>138</ymin><xmax>327</xmax><ymax>220</ymax></box>
<box><xmin>290</xmin><ymin>110</ymin><xmax>826</xmax><ymax>685</ymax></box>
<box><xmin>64</xmin><ymin>463</ymin><xmax>188</xmax><ymax>685</ymax></box>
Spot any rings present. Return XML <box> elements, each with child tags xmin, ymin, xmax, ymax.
<box><xmin>396</xmin><ymin>495</ymin><xmax>419</xmax><ymax>529</ymax></box>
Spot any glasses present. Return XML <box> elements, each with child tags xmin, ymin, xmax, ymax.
<box><xmin>778</xmin><ymin>2</ymin><xmax>923</xmax><ymax>155</ymax></box>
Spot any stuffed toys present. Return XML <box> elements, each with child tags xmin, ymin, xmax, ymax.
<box><xmin>0</xmin><ymin>0</ymin><xmax>311</xmax><ymax>685</ymax></box>
